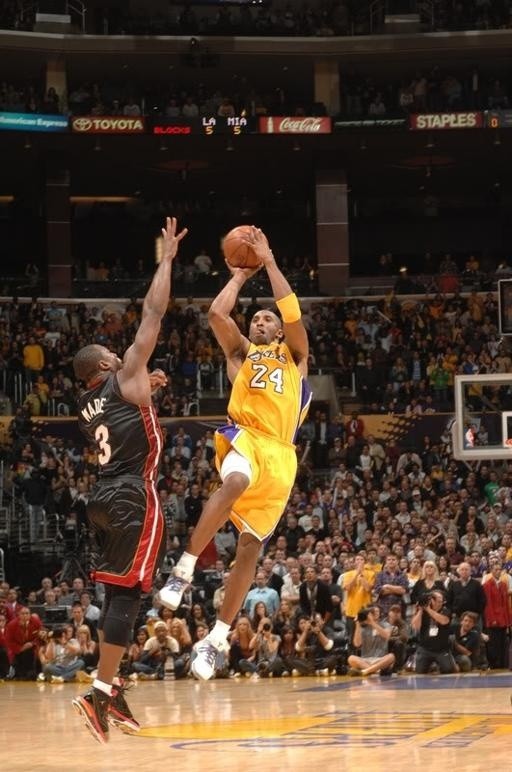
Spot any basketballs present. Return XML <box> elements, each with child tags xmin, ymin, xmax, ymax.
<box><xmin>220</xmin><ymin>223</ymin><xmax>268</xmax><ymax>270</ymax></box>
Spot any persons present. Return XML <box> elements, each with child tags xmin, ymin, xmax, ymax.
<box><xmin>154</xmin><ymin>225</ymin><xmax>313</xmax><ymax>681</ymax></box>
<box><xmin>71</xmin><ymin>253</ymin><xmax>318</xmax><ymax>297</ymax></box>
<box><xmin>374</xmin><ymin>249</ymin><xmax>508</xmax><ymax>295</ymax></box>
<box><xmin>178</xmin><ymin>7</ymin><xmax>386</xmax><ymax>37</ymax></box>
<box><xmin>420</xmin><ymin>8</ymin><xmax>507</xmax><ymax>30</ymax></box>
<box><xmin>2</xmin><ymin>288</ymin><xmax>511</xmax><ymax>683</ymax></box>
<box><xmin>5</xmin><ymin>76</ymin><xmax>326</xmax><ymax>118</ymax></box>
<box><xmin>339</xmin><ymin>67</ymin><xmax>506</xmax><ymax>115</ymax></box>
<box><xmin>26</xmin><ymin>262</ymin><xmax>40</xmax><ymax>289</ymax></box>
<box><xmin>69</xmin><ymin>211</ymin><xmax>193</xmax><ymax>746</ymax></box>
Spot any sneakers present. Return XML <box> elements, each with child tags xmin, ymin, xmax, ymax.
<box><xmin>72</xmin><ymin>686</ymin><xmax>118</xmax><ymax>745</ymax></box>
<box><xmin>48</xmin><ymin>675</ymin><xmax>63</xmax><ymax>684</ymax></box>
<box><xmin>154</xmin><ymin>567</ymin><xmax>195</xmax><ymax>611</ymax></box>
<box><xmin>76</xmin><ymin>670</ymin><xmax>94</xmax><ymax>683</ymax></box>
<box><xmin>229</xmin><ymin>662</ymin><xmax>417</xmax><ymax>680</ymax></box>
<box><xmin>4</xmin><ymin>665</ymin><xmax>15</xmax><ymax>680</ymax></box>
<box><xmin>106</xmin><ymin>678</ymin><xmax>140</xmax><ymax>736</ymax></box>
<box><xmin>35</xmin><ymin>672</ymin><xmax>47</xmax><ymax>682</ymax></box>
<box><xmin>138</xmin><ymin>672</ymin><xmax>150</xmax><ymax>680</ymax></box>
<box><xmin>190</xmin><ymin>634</ymin><xmax>231</xmax><ymax>682</ymax></box>
<box><xmin>127</xmin><ymin>672</ymin><xmax>139</xmax><ymax>681</ymax></box>
